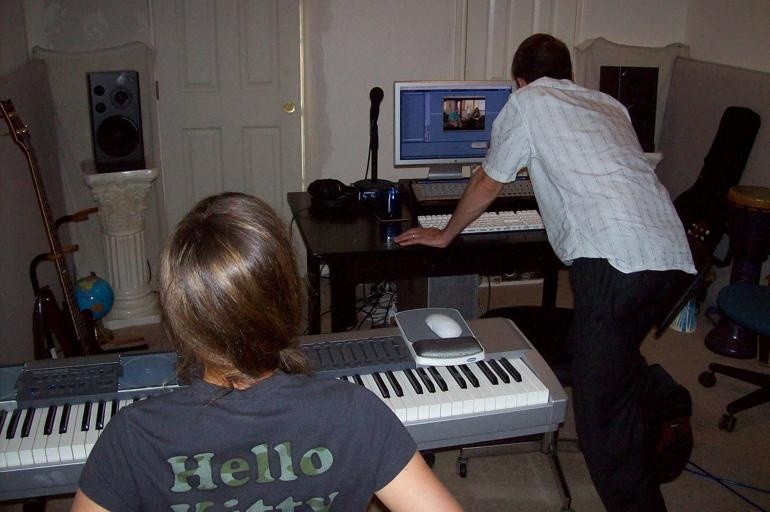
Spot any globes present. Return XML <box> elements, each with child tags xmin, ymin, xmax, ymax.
<box><xmin>75</xmin><ymin>277</ymin><xmax>115</xmax><ymax>345</ymax></box>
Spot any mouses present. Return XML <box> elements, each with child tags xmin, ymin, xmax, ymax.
<box><xmin>425</xmin><ymin>314</ymin><xmax>462</xmax><ymax>339</ymax></box>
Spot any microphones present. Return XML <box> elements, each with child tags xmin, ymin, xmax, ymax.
<box><xmin>369</xmin><ymin>87</ymin><xmax>384</xmax><ymax>129</ymax></box>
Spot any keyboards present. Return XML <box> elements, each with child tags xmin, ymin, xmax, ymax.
<box><xmin>417</xmin><ymin>210</ymin><xmax>544</xmax><ymax>234</ymax></box>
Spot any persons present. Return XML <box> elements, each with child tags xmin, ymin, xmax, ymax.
<box><xmin>471</xmin><ymin>107</ymin><xmax>481</xmax><ymax>121</ymax></box>
<box><xmin>390</xmin><ymin>34</ymin><xmax>700</xmax><ymax>511</ymax></box>
<box><xmin>71</xmin><ymin>190</ymin><xmax>465</xmax><ymax>512</ymax></box>
<box><xmin>447</xmin><ymin>107</ymin><xmax>460</xmax><ymax>129</ymax></box>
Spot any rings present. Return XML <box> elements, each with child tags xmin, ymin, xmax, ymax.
<box><xmin>412</xmin><ymin>233</ymin><xmax>415</xmax><ymax>240</ymax></box>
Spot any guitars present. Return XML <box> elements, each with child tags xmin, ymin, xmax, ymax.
<box><xmin>0</xmin><ymin>97</ymin><xmax>97</xmax><ymax>357</ymax></box>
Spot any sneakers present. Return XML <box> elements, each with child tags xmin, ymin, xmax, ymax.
<box><xmin>651</xmin><ymin>381</ymin><xmax>694</xmax><ymax>483</ymax></box>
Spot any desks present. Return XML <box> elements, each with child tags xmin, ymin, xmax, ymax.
<box><xmin>286</xmin><ymin>172</ymin><xmax>559</xmax><ymax>336</ymax></box>
<box><xmin>83</xmin><ymin>157</ymin><xmax>166</xmax><ymax>334</ymax></box>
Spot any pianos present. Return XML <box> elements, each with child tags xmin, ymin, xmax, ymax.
<box><xmin>0</xmin><ymin>318</ymin><xmax>569</xmax><ymax>498</ymax></box>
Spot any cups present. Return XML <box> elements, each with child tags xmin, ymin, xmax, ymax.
<box><xmin>386</xmin><ymin>187</ymin><xmax>399</xmax><ymax>216</ymax></box>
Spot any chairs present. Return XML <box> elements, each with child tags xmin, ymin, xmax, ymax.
<box><xmin>454</xmin><ymin>302</ymin><xmax>577</xmax><ymax>511</ymax></box>
<box><xmin>699</xmin><ymin>284</ymin><xmax>768</xmax><ymax>433</ymax></box>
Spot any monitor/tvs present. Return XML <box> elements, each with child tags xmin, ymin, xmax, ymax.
<box><xmin>393</xmin><ymin>80</ymin><xmax>513</xmax><ymax>180</ymax></box>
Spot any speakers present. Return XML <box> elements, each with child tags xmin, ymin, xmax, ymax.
<box><xmin>599</xmin><ymin>66</ymin><xmax>659</xmax><ymax>151</ymax></box>
<box><xmin>87</xmin><ymin>70</ymin><xmax>147</xmax><ymax>173</ymax></box>
